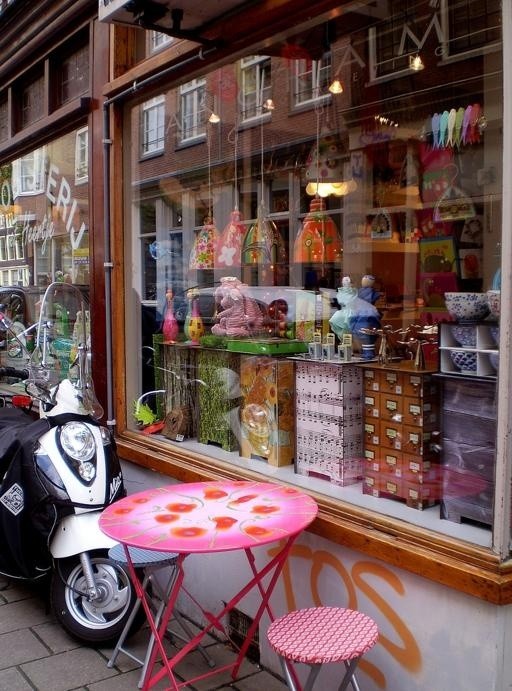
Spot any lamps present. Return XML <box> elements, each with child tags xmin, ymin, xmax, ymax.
<box><xmin>187</xmin><ymin>88</ymin><xmax>341</xmax><ymax>273</ymax></box>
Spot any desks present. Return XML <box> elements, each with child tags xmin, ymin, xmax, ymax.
<box><xmin>98</xmin><ymin>479</ymin><xmax>318</xmax><ymax>691</ymax></box>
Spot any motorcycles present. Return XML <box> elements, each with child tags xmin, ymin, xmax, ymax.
<box><xmin>0</xmin><ymin>281</ymin><xmax>154</xmax><ymax>651</ymax></box>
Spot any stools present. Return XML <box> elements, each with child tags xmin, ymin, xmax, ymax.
<box><xmin>266</xmin><ymin>608</ymin><xmax>379</xmax><ymax>691</ymax></box>
<box><xmin>106</xmin><ymin>542</ymin><xmax>215</xmax><ymax>690</ymax></box>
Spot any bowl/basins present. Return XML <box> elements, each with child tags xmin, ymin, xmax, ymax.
<box><xmin>444</xmin><ymin>290</ymin><xmax>502</xmax><ymax>374</ymax></box>
